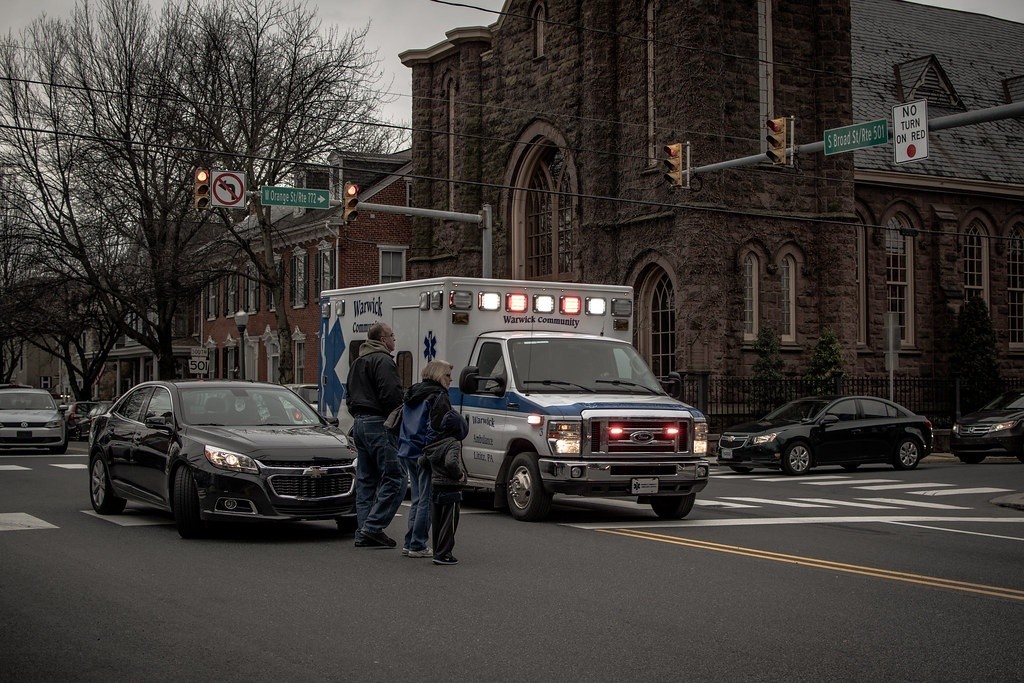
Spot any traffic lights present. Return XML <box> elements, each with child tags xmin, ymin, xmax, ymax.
<box><xmin>765</xmin><ymin>117</ymin><xmax>786</xmax><ymax>167</ymax></box>
<box><xmin>343</xmin><ymin>182</ymin><xmax>359</xmax><ymax>220</ymax></box>
<box><xmin>194</xmin><ymin>168</ymin><xmax>210</xmax><ymax>208</ymax></box>
<box><xmin>662</xmin><ymin>142</ymin><xmax>682</xmax><ymax>187</ymax></box>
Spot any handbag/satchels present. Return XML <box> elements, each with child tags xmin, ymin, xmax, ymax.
<box><xmin>383</xmin><ymin>404</ymin><xmax>405</xmax><ymax>438</ymax></box>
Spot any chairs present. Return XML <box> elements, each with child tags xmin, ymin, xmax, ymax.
<box><xmin>206</xmin><ymin>397</ymin><xmax>226</xmax><ymax>413</ymax></box>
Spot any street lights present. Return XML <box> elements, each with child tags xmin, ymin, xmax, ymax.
<box><xmin>238</xmin><ymin>308</ymin><xmax>249</xmax><ymax>382</ymax></box>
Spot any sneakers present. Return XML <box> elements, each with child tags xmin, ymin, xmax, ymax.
<box><xmin>433</xmin><ymin>556</ymin><xmax>459</xmax><ymax>565</ymax></box>
<box><xmin>402</xmin><ymin>548</ymin><xmax>409</xmax><ymax>555</ymax></box>
<box><xmin>408</xmin><ymin>547</ymin><xmax>434</xmax><ymax>558</ymax></box>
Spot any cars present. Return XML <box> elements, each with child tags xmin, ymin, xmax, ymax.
<box><xmin>716</xmin><ymin>395</ymin><xmax>935</xmax><ymax>477</ymax></box>
<box><xmin>87</xmin><ymin>378</ymin><xmax>358</xmax><ymax>540</ymax></box>
<box><xmin>62</xmin><ymin>400</ymin><xmax>114</xmax><ymax>441</ymax></box>
<box><xmin>0</xmin><ymin>388</ymin><xmax>69</xmax><ymax>454</ymax></box>
<box><xmin>258</xmin><ymin>383</ymin><xmax>336</xmax><ymax>425</ymax></box>
<box><xmin>948</xmin><ymin>389</ymin><xmax>1024</xmax><ymax>464</ymax></box>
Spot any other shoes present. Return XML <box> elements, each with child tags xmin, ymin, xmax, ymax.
<box><xmin>360</xmin><ymin>530</ymin><xmax>396</xmax><ymax>547</ymax></box>
<box><xmin>356</xmin><ymin>540</ymin><xmax>385</xmax><ymax>547</ymax></box>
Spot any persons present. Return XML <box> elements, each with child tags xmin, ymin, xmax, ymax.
<box><xmin>396</xmin><ymin>360</ymin><xmax>468</xmax><ymax>558</ymax></box>
<box><xmin>418</xmin><ymin>437</ymin><xmax>467</xmax><ymax>565</ymax></box>
<box><xmin>346</xmin><ymin>323</ymin><xmax>408</xmax><ymax>549</ymax></box>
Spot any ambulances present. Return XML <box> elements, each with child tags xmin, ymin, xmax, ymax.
<box><xmin>317</xmin><ymin>275</ymin><xmax>710</xmax><ymax>522</ymax></box>
